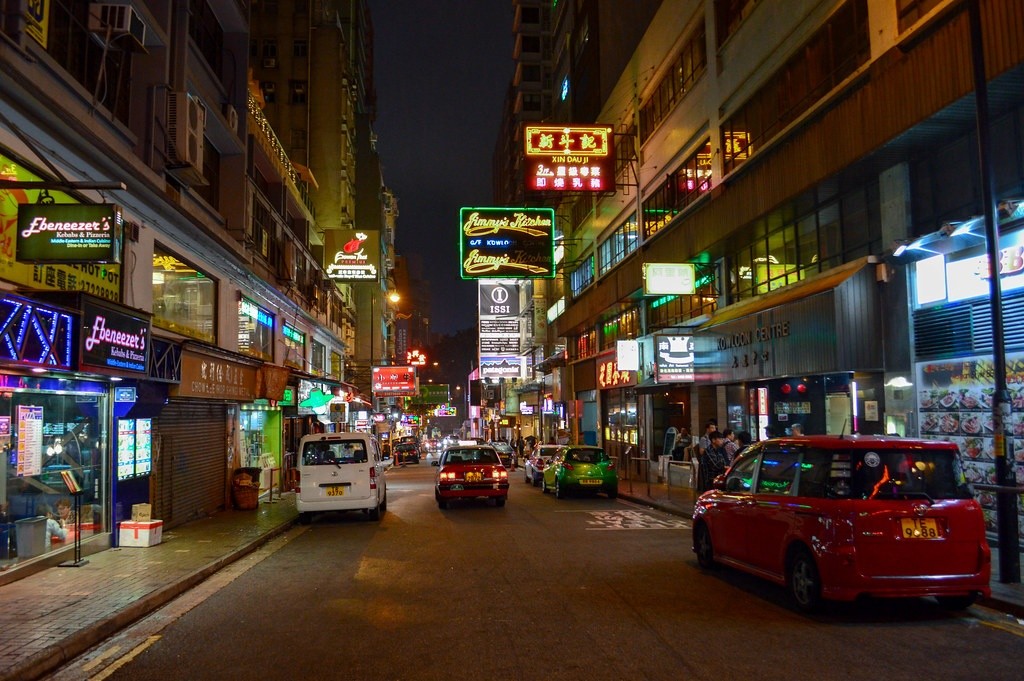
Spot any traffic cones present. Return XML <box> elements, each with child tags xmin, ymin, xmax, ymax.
<box><xmin>510</xmin><ymin>459</ymin><xmax>515</xmax><ymax>472</ymax></box>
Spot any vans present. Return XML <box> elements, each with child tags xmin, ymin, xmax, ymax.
<box><xmin>295</xmin><ymin>432</ymin><xmax>388</xmax><ymax>522</ymax></box>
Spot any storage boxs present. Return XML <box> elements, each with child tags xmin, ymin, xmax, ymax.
<box><xmin>119</xmin><ymin>519</ymin><xmax>164</xmax><ymax>548</ymax></box>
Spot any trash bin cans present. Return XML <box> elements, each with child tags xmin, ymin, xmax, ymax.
<box><xmin>232</xmin><ymin>466</ymin><xmax>262</xmax><ymax>511</ymax></box>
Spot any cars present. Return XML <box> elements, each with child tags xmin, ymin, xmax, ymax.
<box><xmin>487</xmin><ymin>442</ymin><xmax>518</xmax><ymax>467</ymax></box>
<box><xmin>524</xmin><ymin>444</ymin><xmax>569</xmax><ymax>487</ymax></box>
<box><xmin>391</xmin><ymin>434</ymin><xmax>486</xmax><ymax>465</ymax></box>
<box><xmin>431</xmin><ymin>445</ymin><xmax>510</xmax><ymax>509</ymax></box>
<box><xmin>542</xmin><ymin>445</ymin><xmax>618</xmax><ymax>499</ymax></box>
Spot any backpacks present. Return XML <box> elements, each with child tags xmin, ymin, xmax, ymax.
<box><xmin>719</xmin><ymin>440</ymin><xmax>730</xmax><ymax>466</ymax></box>
<box><xmin>693</xmin><ymin>435</ymin><xmax>708</xmax><ymax>463</ymax></box>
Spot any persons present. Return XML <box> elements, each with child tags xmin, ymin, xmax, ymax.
<box><xmin>790</xmin><ymin>423</ymin><xmax>804</xmax><ymax>436</ymax></box>
<box><xmin>35</xmin><ymin>505</ymin><xmax>69</xmax><ymax>553</ymax></box>
<box><xmin>671</xmin><ymin>427</ymin><xmax>692</xmax><ymax>461</ymax></box>
<box><xmin>699</xmin><ymin>422</ymin><xmax>717</xmax><ymax>456</ymax></box>
<box><xmin>702</xmin><ymin>431</ymin><xmax>730</xmax><ymax>491</ymax></box>
<box><xmin>54</xmin><ymin>499</ymin><xmax>76</xmax><ymax>525</ymax></box>
<box><xmin>731</xmin><ymin>430</ymin><xmax>752</xmax><ymax>467</ymax></box>
<box><xmin>493</xmin><ymin>436</ymin><xmax>556</xmax><ymax>458</ymax></box>
<box><xmin>721</xmin><ymin>429</ymin><xmax>738</xmax><ymax>462</ymax></box>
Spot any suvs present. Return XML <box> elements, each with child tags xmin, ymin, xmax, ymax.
<box><xmin>690</xmin><ymin>418</ymin><xmax>995</xmax><ymax>619</ymax></box>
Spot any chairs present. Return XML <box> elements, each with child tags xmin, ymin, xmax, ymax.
<box><xmin>479</xmin><ymin>454</ymin><xmax>491</xmax><ymax>462</ymax></box>
<box><xmin>449</xmin><ymin>455</ymin><xmax>463</xmax><ymax>464</ymax></box>
